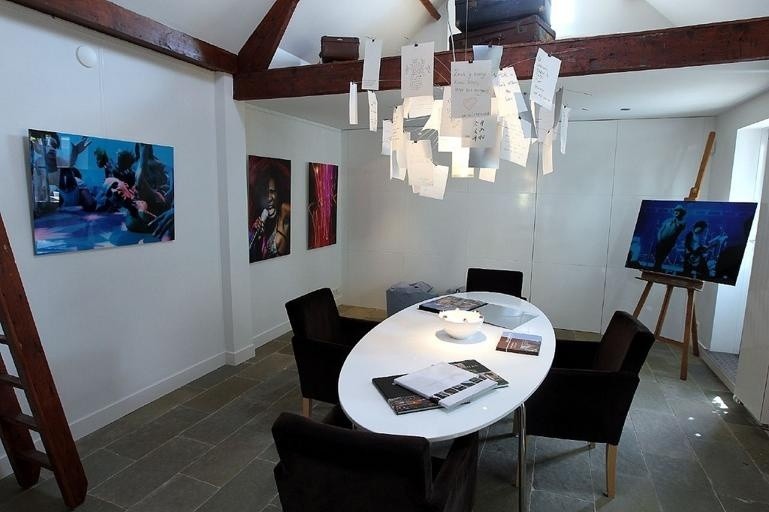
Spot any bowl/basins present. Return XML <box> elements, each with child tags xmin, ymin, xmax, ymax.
<box><xmin>437</xmin><ymin>309</ymin><xmax>484</xmax><ymax>340</ymax></box>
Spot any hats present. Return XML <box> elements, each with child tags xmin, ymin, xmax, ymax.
<box><xmin>694</xmin><ymin>221</ymin><xmax>707</xmax><ymax>229</ymax></box>
<box><xmin>673</xmin><ymin>205</ymin><xmax>686</xmax><ymax>217</ymax></box>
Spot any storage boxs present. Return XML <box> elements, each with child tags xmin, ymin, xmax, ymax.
<box><xmin>319</xmin><ymin>34</ymin><xmax>360</xmax><ymax>63</ymax></box>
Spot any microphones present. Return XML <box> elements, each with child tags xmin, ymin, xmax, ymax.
<box><xmin>249</xmin><ymin>208</ymin><xmax>268</xmax><ymax>249</ymax></box>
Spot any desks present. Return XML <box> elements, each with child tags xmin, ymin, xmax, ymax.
<box><xmin>333</xmin><ymin>288</ymin><xmax>558</xmax><ymax>511</ymax></box>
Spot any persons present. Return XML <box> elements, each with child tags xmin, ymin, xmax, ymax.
<box><xmin>651</xmin><ymin>203</ymin><xmax>710</xmax><ymax>278</ymax></box>
<box><xmin>30</xmin><ymin>130</ymin><xmax>174</xmax><ymax>242</ymax></box>
<box><xmin>250</xmin><ymin>158</ymin><xmax>291</xmax><ymax>262</ymax></box>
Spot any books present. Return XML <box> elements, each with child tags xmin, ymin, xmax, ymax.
<box><xmin>372</xmin><ymin>295</ymin><xmax>542</xmax><ymax>415</ymax></box>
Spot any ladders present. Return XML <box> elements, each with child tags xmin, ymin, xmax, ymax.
<box><xmin>0</xmin><ymin>213</ymin><xmax>89</xmax><ymax>509</ymax></box>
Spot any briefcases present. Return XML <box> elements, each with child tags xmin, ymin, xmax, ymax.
<box><xmin>449</xmin><ymin>15</ymin><xmax>555</xmax><ymax>51</ymax></box>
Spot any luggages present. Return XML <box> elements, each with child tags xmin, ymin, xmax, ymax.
<box><xmin>455</xmin><ymin>0</ymin><xmax>551</xmax><ymax>32</ymax></box>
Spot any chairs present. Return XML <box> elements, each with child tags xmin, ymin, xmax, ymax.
<box><xmin>285</xmin><ymin>288</ymin><xmax>390</xmax><ymax>421</ymax></box>
<box><xmin>271</xmin><ymin>399</ymin><xmax>488</xmax><ymax>510</ymax></box>
<box><xmin>512</xmin><ymin>308</ymin><xmax>657</xmax><ymax>498</ymax></box>
<box><xmin>464</xmin><ymin>263</ymin><xmax>526</xmax><ymax>297</ymax></box>
